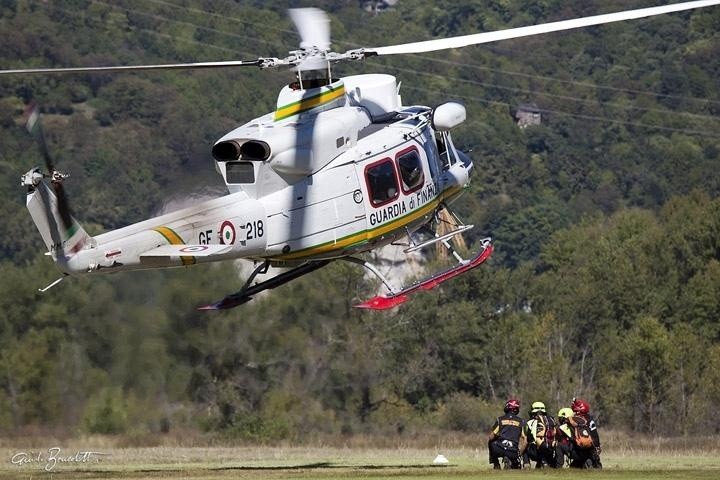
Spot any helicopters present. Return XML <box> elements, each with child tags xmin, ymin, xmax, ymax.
<box><xmin>0</xmin><ymin>1</ymin><xmax>719</xmax><ymax>309</ymax></box>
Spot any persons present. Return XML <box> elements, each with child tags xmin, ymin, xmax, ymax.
<box><xmin>516</xmin><ymin>401</ymin><xmax>563</xmax><ymax>470</ymax></box>
<box><xmin>553</xmin><ymin>407</ymin><xmax>603</xmax><ymax>469</ymax></box>
<box><xmin>488</xmin><ymin>399</ymin><xmax>536</xmax><ymax>470</ymax></box>
<box><xmin>570</xmin><ymin>400</ymin><xmax>602</xmax><ymax>456</ymax></box>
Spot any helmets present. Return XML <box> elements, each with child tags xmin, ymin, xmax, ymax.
<box><xmin>530</xmin><ymin>401</ymin><xmax>547</xmax><ymax>413</ymax></box>
<box><xmin>504</xmin><ymin>399</ymin><xmax>520</xmax><ymax>415</ymax></box>
<box><xmin>571</xmin><ymin>399</ymin><xmax>590</xmax><ymax>413</ymax></box>
<box><xmin>558</xmin><ymin>408</ymin><xmax>574</xmax><ymax>419</ymax></box>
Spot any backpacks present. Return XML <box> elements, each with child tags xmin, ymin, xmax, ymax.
<box><xmin>568</xmin><ymin>417</ymin><xmax>593</xmax><ymax>449</ymax></box>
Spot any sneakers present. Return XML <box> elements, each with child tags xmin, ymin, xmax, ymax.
<box><xmin>585</xmin><ymin>453</ymin><xmax>603</xmax><ymax>469</ymax></box>
<box><xmin>494</xmin><ymin>463</ymin><xmax>531</xmax><ymax>470</ymax></box>
